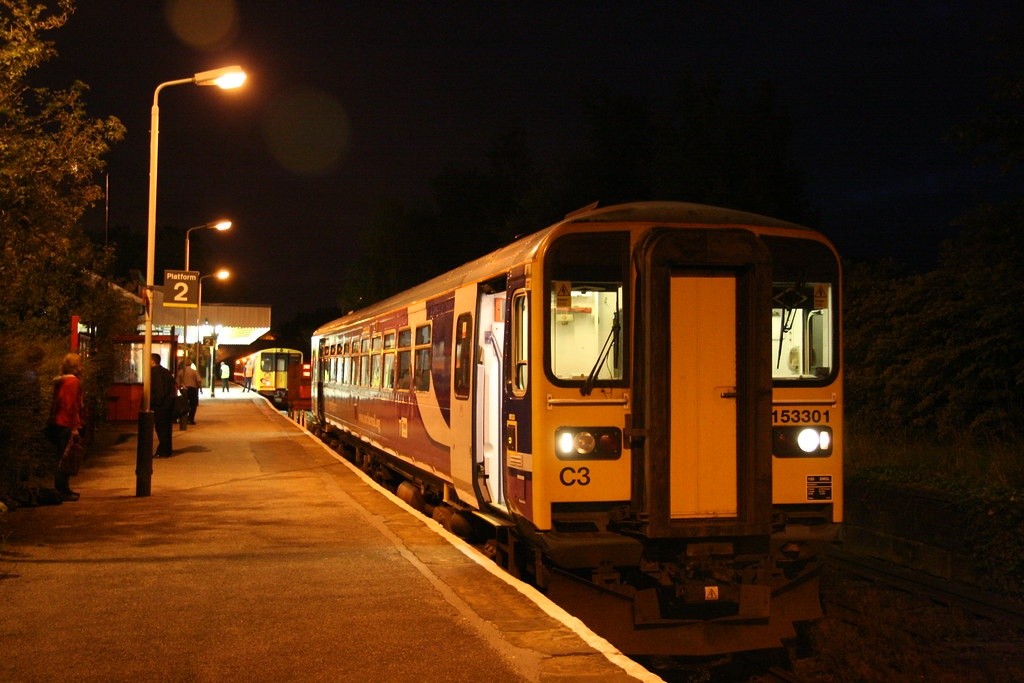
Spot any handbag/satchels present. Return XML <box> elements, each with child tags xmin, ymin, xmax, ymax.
<box><xmin>58</xmin><ymin>435</ymin><xmax>87</xmax><ymax>476</ymax></box>
<box><xmin>171</xmin><ymin>394</ymin><xmax>189</xmax><ymax>418</ymax></box>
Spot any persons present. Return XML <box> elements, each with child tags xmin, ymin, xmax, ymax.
<box><xmin>242</xmin><ymin>361</ymin><xmax>254</xmax><ymax>392</ymax></box>
<box><xmin>175</xmin><ymin>359</ymin><xmax>202</xmax><ymax>425</ymax></box>
<box><xmin>140</xmin><ymin>353</ymin><xmax>179</xmax><ymax>459</ymax></box>
<box><xmin>219</xmin><ymin>361</ymin><xmax>230</xmax><ymax>392</ymax></box>
<box><xmin>47</xmin><ymin>353</ymin><xmax>84</xmax><ymax>501</ymax></box>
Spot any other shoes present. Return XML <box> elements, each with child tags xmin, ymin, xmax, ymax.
<box><xmin>153</xmin><ymin>452</ymin><xmax>171</xmax><ymax>458</ymax></box>
<box><xmin>56</xmin><ymin>488</ymin><xmax>80</xmax><ymax>501</ymax></box>
<box><xmin>188</xmin><ymin>420</ymin><xmax>195</xmax><ymax>425</ymax></box>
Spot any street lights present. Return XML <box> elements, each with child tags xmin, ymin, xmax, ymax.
<box><xmin>135</xmin><ymin>64</ymin><xmax>248</xmax><ymax>497</ymax></box>
<box><xmin>179</xmin><ymin>218</ymin><xmax>232</xmax><ymax>431</ymax></box>
<box><xmin>195</xmin><ymin>270</ymin><xmax>229</xmax><ymax>370</ymax></box>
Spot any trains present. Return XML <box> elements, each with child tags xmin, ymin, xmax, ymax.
<box><xmin>233</xmin><ymin>347</ymin><xmax>303</xmax><ymax>406</ymax></box>
<box><xmin>287</xmin><ymin>201</ymin><xmax>842</xmax><ymax>656</ymax></box>
